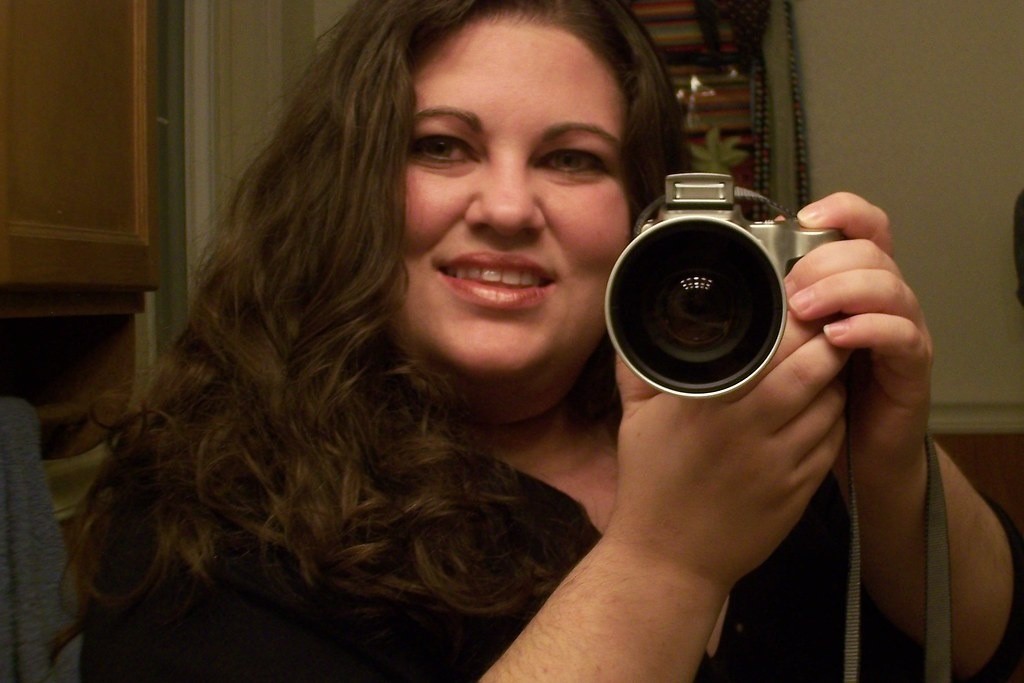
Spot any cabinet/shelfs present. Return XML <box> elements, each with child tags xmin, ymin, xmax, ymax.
<box><xmin>0</xmin><ymin>0</ymin><xmax>160</xmax><ymax>317</ymax></box>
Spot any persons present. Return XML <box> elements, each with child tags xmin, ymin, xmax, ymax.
<box><xmin>44</xmin><ymin>1</ymin><xmax>1024</xmax><ymax>683</ymax></box>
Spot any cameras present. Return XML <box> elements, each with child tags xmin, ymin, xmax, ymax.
<box><xmin>604</xmin><ymin>172</ymin><xmax>846</xmax><ymax>399</ymax></box>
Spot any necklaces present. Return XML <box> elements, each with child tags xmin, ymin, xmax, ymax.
<box><xmin>757</xmin><ymin>0</ymin><xmax>810</xmax><ymax>225</ymax></box>
